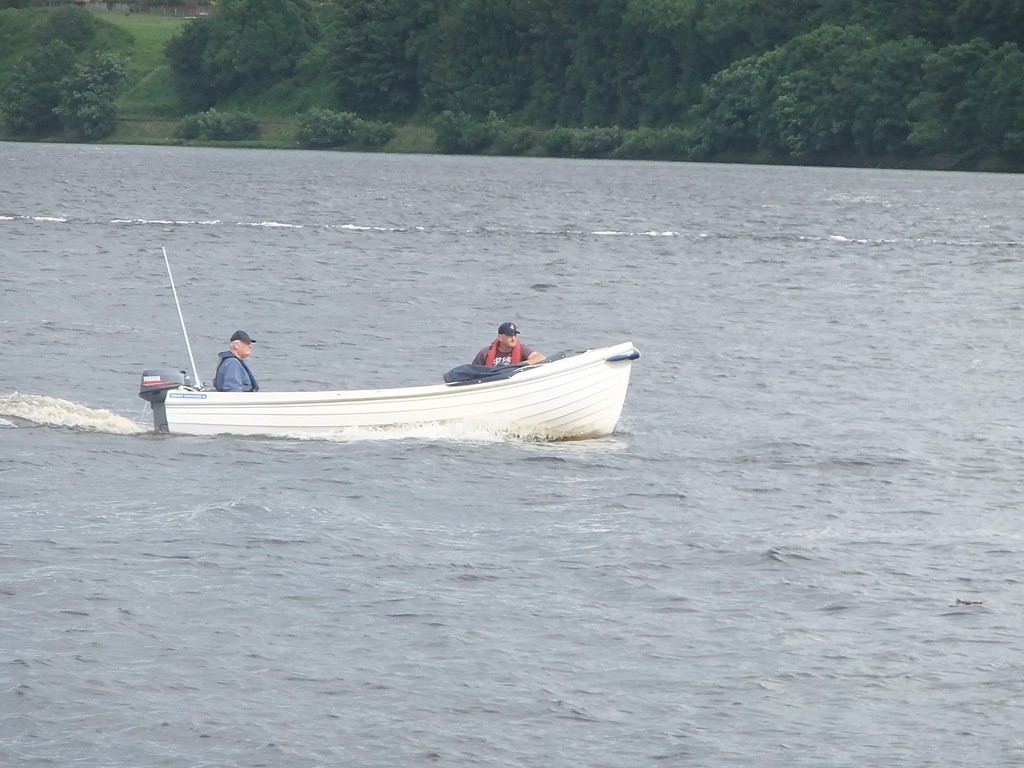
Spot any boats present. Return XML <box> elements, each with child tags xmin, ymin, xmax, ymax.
<box><xmin>139</xmin><ymin>245</ymin><xmax>641</xmax><ymax>441</ymax></box>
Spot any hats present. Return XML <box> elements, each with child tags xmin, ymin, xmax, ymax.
<box><xmin>498</xmin><ymin>321</ymin><xmax>520</xmax><ymax>335</ymax></box>
<box><xmin>230</xmin><ymin>330</ymin><xmax>257</xmax><ymax>344</ymax></box>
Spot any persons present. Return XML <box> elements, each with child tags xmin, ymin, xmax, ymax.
<box><xmin>471</xmin><ymin>322</ymin><xmax>546</xmax><ymax>369</ymax></box>
<box><xmin>213</xmin><ymin>330</ymin><xmax>259</xmax><ymax>392</ymax></box>
